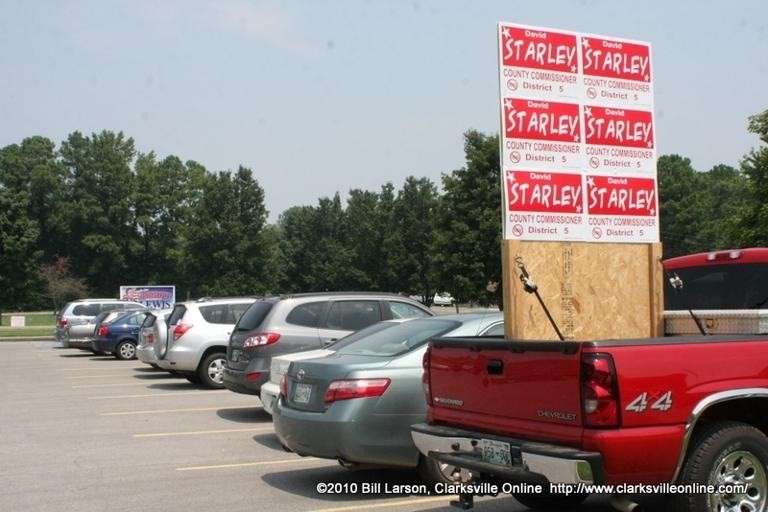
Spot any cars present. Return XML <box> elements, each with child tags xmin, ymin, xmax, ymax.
<box><xmin>58</xmin><ymin>289</ymin><xmax>514</xmax><ymax>484</ymax></box>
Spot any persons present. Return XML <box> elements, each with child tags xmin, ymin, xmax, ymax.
<box><xmin>125</xmin><ymin>288</ymin><xmax>135</xmax><ymax>300</ymax></box>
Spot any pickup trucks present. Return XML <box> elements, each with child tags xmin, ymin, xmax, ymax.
<box><xmin>411</xmin><ymin>239</ymin><xmax>768</xmax><ymax>512</ymax></box>
